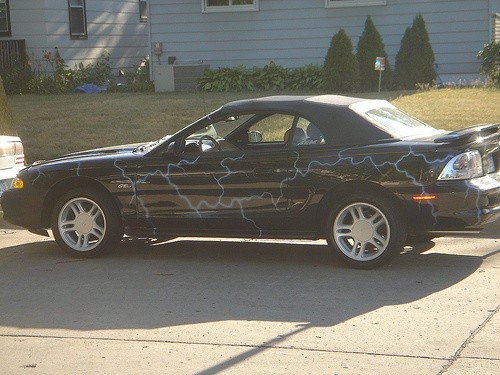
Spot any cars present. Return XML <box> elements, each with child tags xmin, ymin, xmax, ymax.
<box><xmin>0</xmin><ymin>135</ymin><xmax>26</xmax><ymax>180</ymax></box>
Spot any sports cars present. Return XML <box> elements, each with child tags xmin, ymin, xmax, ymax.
<box><xmin>0</xmin><ymin>93</ymin><xmax>500</xmax><ymax>270</ymax></box>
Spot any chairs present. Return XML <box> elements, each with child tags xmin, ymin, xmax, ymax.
<box><xmin>284</xmin><ymin>122</ymin><xmax>321</xmax><ymax>146</ymax></box>
